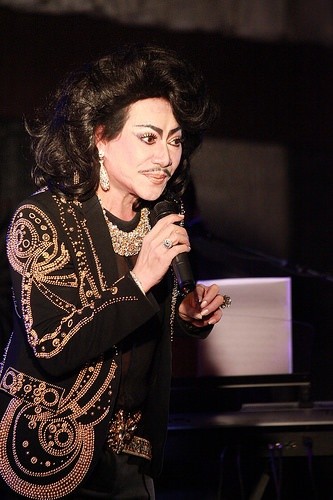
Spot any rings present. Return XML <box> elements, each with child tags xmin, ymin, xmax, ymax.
<box><xmin>221</xmin><ymin>295</ymin><xmax>232</xmax><ymax>309</ymax></box>
<box><xmin>164</xmin><ymin>239</ymin><xmax>173</xmax><ymax>249</ymax></box>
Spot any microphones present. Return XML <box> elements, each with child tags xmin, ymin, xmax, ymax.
<box><xmin>154</xmin><ymin>201</ymin><xmax>196</xmax><ymax>294</ymax></box>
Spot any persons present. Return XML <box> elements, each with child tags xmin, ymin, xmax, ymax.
<box><xmin>0</xmin><ymin>40</ymin><xmax>224</xmax><ymax>500</ymax></box>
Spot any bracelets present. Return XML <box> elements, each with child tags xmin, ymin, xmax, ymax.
<box><xmin>130</xmin><ymin>271</ymin><xmax>146</xmax><ymax>296</ymax></box>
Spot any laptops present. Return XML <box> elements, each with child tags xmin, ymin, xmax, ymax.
<box><xmin>193</xmin><ymin>274</ymin><xmax>300</xmax><ymax>383</ymax></box>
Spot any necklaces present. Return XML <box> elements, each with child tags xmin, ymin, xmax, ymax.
<box><xmin>95</xmin><ymin>194</ymin><xmax>152</xmax><ymax>257</ymax></box>
